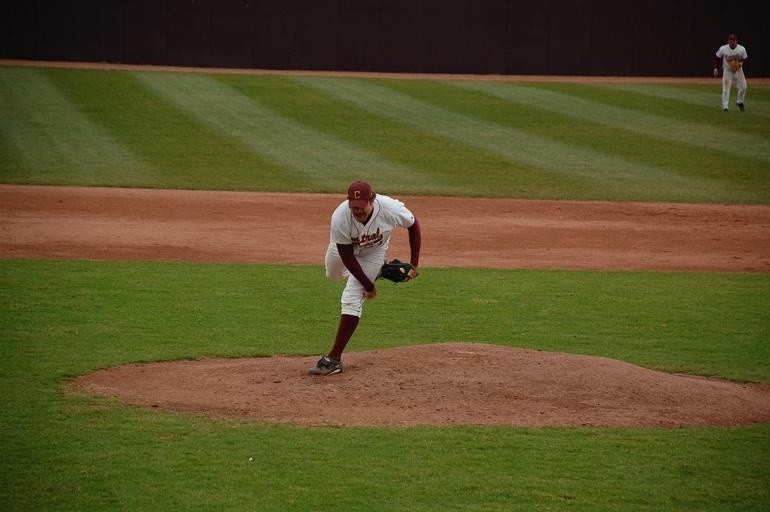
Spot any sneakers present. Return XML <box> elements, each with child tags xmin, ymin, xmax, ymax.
<box><xmin>308</xmin><ymin>355</ymin><xmax>342</xmax><ymax>376</ymax></box>
<box><xmin>736</xmin><ymin>102</ymin><xmax>745</xmax><ymax>111</ymax></box>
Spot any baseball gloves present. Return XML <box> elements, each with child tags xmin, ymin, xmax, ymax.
<box><xmin>379</xmin><ymin>258</ymin><xmax>419</xmax><ymax>282</ymax></box>
<box><xmin>729</xmin><ymin>63</ymin><xmax>741</xmax><ymax>74</ymax></box>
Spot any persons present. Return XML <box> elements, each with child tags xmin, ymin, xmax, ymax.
<box><xmin>713</xmin><ymin>34</ymin><xmax>748</xmax><ymax>112</ymax></box>
<box><xmin>308</xmin><ymin>180</ymin><xmax>421</xmax><ymax>376</ymax></box>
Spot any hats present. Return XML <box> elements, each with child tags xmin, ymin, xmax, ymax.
<box><xmin>348</xmin><ymin>182</ymin><xmax>372</xmax><ymax>208</ymax></box>
